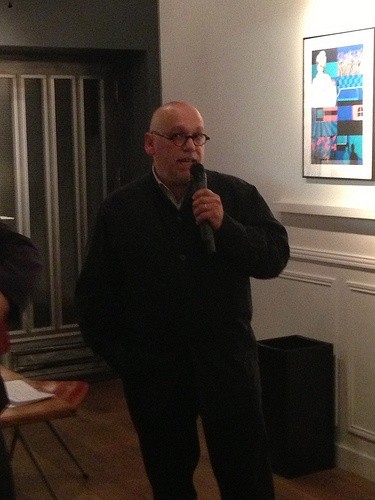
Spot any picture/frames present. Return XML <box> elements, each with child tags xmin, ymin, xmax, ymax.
<box><xmin>303</xmin><ymin>27</ymin><xmax>375</xmax><ymax>181</ymax></box>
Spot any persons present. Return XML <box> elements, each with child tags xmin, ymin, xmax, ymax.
<box><xmin>0</xmin><ymin>219</ymin><xmax>46</xmax><ymax>500</ymax></box>
<box><xmin>73</xmin><ymin>102</ymin><xmax>291</xmax><ymax>500</ymax></box>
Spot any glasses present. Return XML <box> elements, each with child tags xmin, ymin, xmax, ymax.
<box><xmin>151</xmin><ymin>130</ymin><xmax>210</xmax><ymax>146</ymax></box>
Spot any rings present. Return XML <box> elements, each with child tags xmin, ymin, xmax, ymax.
<box><xmin>204</xmin><ymin>204</ymin><xmax>209</xmax><ymax>211</ymax></box>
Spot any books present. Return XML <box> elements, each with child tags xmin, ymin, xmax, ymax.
<box><xmin>4</xmin><ymin>380</ymin><xmax>54</xmax><ymax>408</ymax></box>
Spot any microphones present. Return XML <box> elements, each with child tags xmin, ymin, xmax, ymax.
<box><xmin>189</xmin><ymin>162</ymin><xmax>214</xmax><ymax>242</ymax></box>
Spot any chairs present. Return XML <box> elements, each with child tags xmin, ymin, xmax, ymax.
<box><xmin>0</xmin><ymin>350</ymin><xmax>91</xmax><ymax>500</ymax></box>
